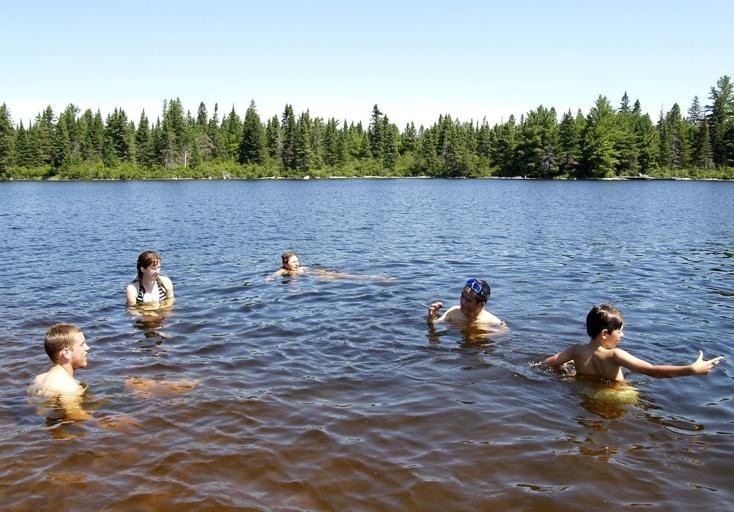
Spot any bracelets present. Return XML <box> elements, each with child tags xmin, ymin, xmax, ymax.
<box><xmin>429</xmin><ymin>315</ymin><xmax>434</xmax><ymax>318</ymax></box>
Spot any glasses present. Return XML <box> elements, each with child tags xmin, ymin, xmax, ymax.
<box><xmin>466</xmin><ymin>278</ymin><xmax>488</xmax><ymax>298</ymax></box>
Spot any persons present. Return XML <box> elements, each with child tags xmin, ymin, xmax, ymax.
<box><xmin>266</xmin><ymin>252</ymin><xmax>311</xmax><ymax>278</ymax></box>
<box><xmin>125</xmin><ymin>250</ymin><xmax>174</xmax><ymax>306</ymax></box>
<box><xmin>28</xmin><ymin>323</ymin><xmax>95</xmax><ymax>422</ymax></box>
<box><xmin>426</xmin><ymin>278</ymin><xmax>510</xmax><ymax>342</ymax></box>
<box><xmin>538</xmin><ymin>304</ymin><xmax>724</xmax><ymax>381</ymax></box>
